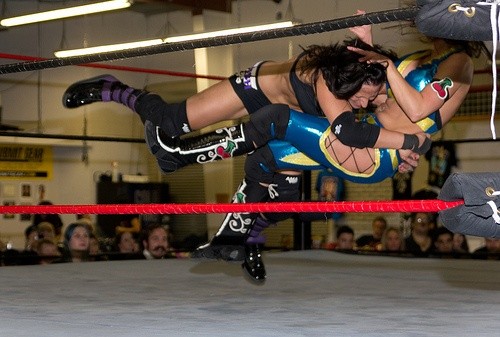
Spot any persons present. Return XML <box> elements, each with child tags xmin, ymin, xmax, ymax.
<box><xmin>0</xmin><ymin>189</ymin><xmax>500</xmax><ymax>267</ymax></box>
<box><xmin>62</xmin><ymin>37</ymin><xmax>433</xmax><ymax>285</ymax></box>
<box><xmin>145</xmin><ymin>0</ymin><xmax>482</xmax><ymax>262</ymax></box>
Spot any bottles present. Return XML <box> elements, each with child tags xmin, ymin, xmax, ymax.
<box><xmin>111</xmin><ymin>160</ymin><xmax>119</xmax><ymax>182</ymax></box>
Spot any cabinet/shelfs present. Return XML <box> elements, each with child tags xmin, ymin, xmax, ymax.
<box><xmin>97</xmin><ymin>181</ymin><xmax>169</xmax><ymax>237</ymax></box>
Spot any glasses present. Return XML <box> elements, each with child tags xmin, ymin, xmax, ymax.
<box><xmin>413</xmin><ymin>218</ymin><xmax>430</xmax><ymax>224</ymax></box>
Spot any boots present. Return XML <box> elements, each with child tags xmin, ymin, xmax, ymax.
<box><xmin>194</xmin><ymin>176</ymin><xmax>278</xmax><ymax>263</ymax></box>
<box><xmin>62</xmin><ymin>75</ymin><xmax>151</xmax><ymax>112</ymax></box>
<box><xmin>144</xmin><ymin>120</ymin><xmax>255</xmax><ymax>173</ymax></box>
<box><xmin>241</xmin><ymin>213</ymin><xmax>270</xmax><ymax>282</ymax></box>
